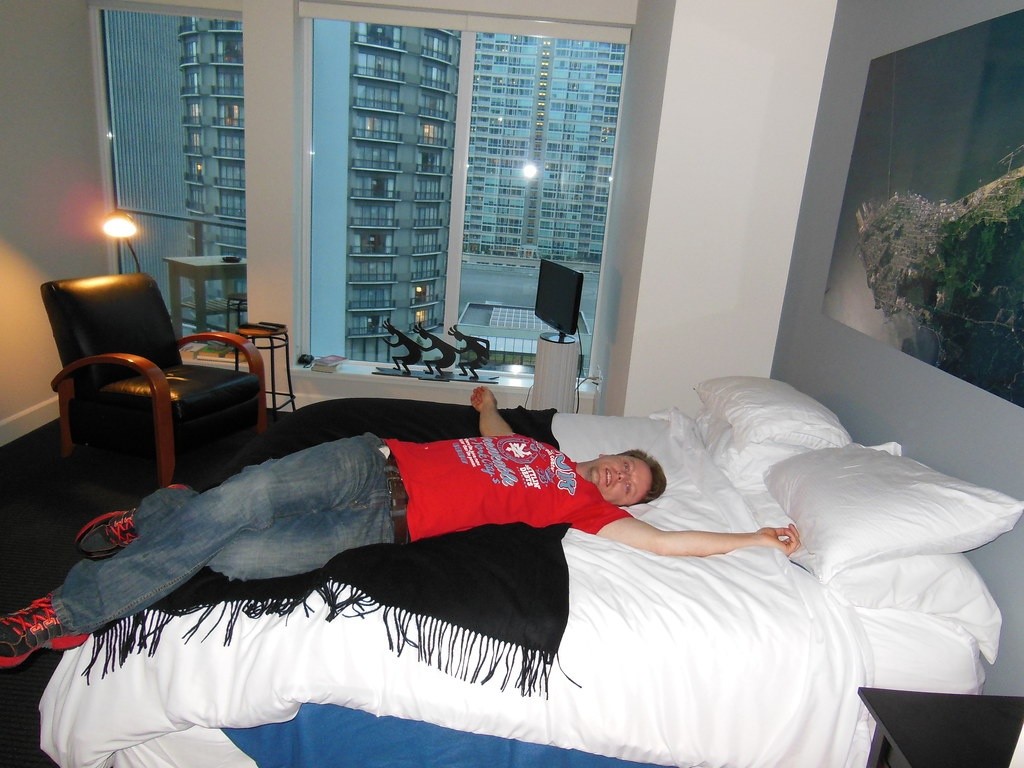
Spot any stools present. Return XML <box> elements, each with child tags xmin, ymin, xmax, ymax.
<box><xmin>225</xmin><ymin>293</ymin><xmax>247</xmax><ymax>344</ymax></box>
<box><xmin>235</xmin><ymin>323</ymin><xmax>296</xmax><ymax>422</ymax></box>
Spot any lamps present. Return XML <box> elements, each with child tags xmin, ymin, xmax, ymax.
<box><xmin>102</xmin><ymin>212</ymin><xmax>141</xmax><ymax>274</ymax></box>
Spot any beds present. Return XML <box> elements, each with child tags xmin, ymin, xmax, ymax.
<box><xmin>36</xmin><ymin>406</ymin><xmax>985</xmax><ymax>768</ymax></box>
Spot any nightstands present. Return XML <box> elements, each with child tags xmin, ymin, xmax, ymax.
<box><xmin>858</xmin><ymin>687</ymin><xmax>1024</xmax><ymax>768</ymax></box>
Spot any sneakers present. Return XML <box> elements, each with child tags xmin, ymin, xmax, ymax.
<box><xmin>75</xmin><ymin>484</ymin><xmax>189</xmax><ymax>559</ymax></box>
<box><xmin>0</xmin><ymin>591</ymin><xmax>89</xmax><ymax>668</ymax></box>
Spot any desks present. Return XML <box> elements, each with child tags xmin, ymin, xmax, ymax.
<box><xmin>162</xmin><ymin>255</ymin><xmax>247</xmax><ymax>338</ymax></box>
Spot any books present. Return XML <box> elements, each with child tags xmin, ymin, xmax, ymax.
<box><xmin>312</xmin><ymin>355</ymin><xmax>348</xmax><ymax>373</ymax></box>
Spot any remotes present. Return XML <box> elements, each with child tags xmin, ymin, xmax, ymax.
<box><xmin>222</xmin><ymin>256</ymin><xmax>241</xmax><ymax>263</ymax></box>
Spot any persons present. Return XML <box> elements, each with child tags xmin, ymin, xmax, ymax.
<box><xmin>1</xmin><ymin>386</ymin><xmax>800</xmax><ymax>669</ymax></box>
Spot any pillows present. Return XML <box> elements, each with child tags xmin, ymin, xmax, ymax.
<box><xmin>739</xmin><ymin>484</ymin><xmax>1003</xmax><ymax>665</ymax></box>
<box><xmin>761</xmin><ymin>441</ymin><xmax>1024</xmax><ymax>588</ymax></box>
<box><xmin>693</xmin><ymin>375</ymin><xmax>851</xmax><ymax>460</ymax></box>
<box><xmin>694</xmin><ymin>409</ymin><xmax>814</xmax><ymax>491</ymax></box>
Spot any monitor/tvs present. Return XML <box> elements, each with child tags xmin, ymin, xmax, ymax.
<box><xmin>534</xmin><ymin>258</ymin><xmax>584</xmax><ymax>343</ymax></box>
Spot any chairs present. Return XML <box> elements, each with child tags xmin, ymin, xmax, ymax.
<box><xmin>40</xmin><ymin>273</ymin><xmax>267</xmax><ymax>488</ymax></box>
